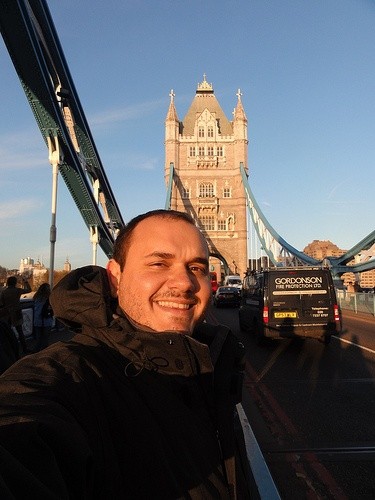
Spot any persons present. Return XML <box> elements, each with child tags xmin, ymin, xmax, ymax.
<box><xmin>348</xmin><ymin>282</ymin><xmax>355</xmax><ymax>306</ymax></box>
<box><xmin>0</xmin><ymin>208</ymin><xmax>247</xmax><ymax>500</ymax></box>
<box><xmin>32</xmin><ymin>282</ymin><xmax>55</xmax><ymax>349</ymax></box>
<box><xmin>0</xmin><ymin>306</ymin><xmax>20</xmax><ymax>375</ymax></box>
<box><xmin>353</xmin><ymin>281</ymin><xmax>362</xmax><ymax>305</ymax></box>
<box><xmin>0</xmin><ymin>276</ymin><xmax>32</xmax><ymax>352</ymax></box>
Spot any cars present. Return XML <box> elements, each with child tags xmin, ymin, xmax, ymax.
<box><xmin>212</xmin><ymin>286</ymin><xmax>240</xmax><ymax>309</ymax></box>
<box><xmin>209</xmin><ymin>272</ymin><xmax>218</xmax><ymax>294</ymax></box>
<box><xmin>223</xmin><ymin>275</ymin><xmax>243</xmax><ymax>293</ymax></box>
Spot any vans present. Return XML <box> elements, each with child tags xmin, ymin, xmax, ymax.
<box><xmin>238</xmin><ymin>266</ymin><xmax>342</xmax><ymax>341</ymax></box>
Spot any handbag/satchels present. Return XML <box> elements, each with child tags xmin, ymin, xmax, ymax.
<box><xmin>40</xmin><ymin>299</ymin><xmax>54</xmax><ymax>319</ymax></box>
